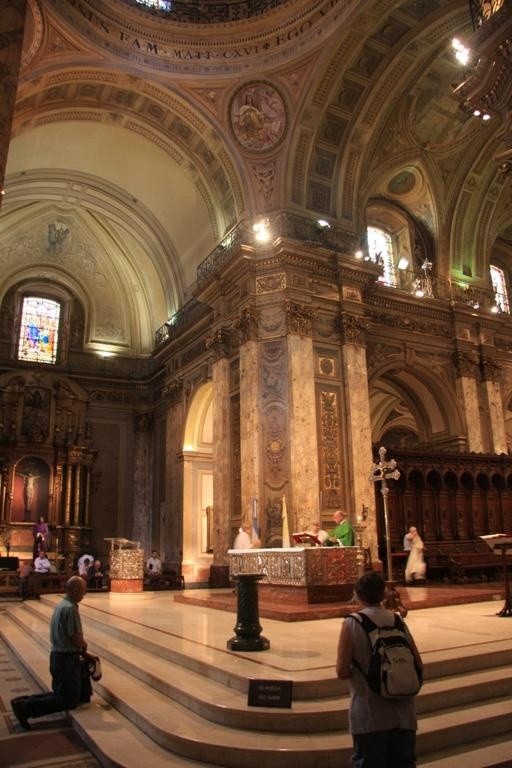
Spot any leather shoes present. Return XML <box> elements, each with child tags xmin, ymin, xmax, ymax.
<box><xmin>12</xmin><ymin>699</ymin><xmax>30</xmax><ymax>729</ymax></box>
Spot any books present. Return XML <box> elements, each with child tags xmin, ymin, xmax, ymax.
<box><xmin>292</xmin><ymin>531</ymin><xmax>321</xmax><ymax>544</ymax></box>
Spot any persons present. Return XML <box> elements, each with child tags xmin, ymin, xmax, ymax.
<box><xmin>88</xmin><ymin>560</ymin><xmax>104</xmax><ymax>588</ymax></box>
<box><xmin>33</xmin><ymin>550</ymin><xmax>52</xmax><ymax>586</ymax></box>
<box><xmin>232</xmin><ymin>520</ymin><xmax>262</xmax><ymax>549</ymax></box>
<box><xmin>320</xmin><ymin>510</ymin><xmax>355</xmax><ymax>546</ymax></box>
<box><xmin>16</xmin><ymin>470</ymin><xmax>42</xmax><ymax>513</ymax></box>
<box><xmin>78</xmin><ymin>552</ymin><xmax>95</xmax><ymax>568</ymax></box>
<box><xmin>16</xmin><ymin>560</ymin><xmax>33</xmax><ymax>601</ymax></box>
<box><xmin>78</xmin><ymin>558</ymin><xmax>90</xmax><ymax>576</ymax></box>
<box><xmin>333</xmin><ymin>571</ymin><xmax>426</xmax><ymax>768</ymax></box>
<box><xmin>33</xmin><ymin>514</ymin><xmax>49</xmax><ymax>560</ymax></box>
<box><xmin>402</xmin><ymin>526</ymin><xmax>427</xmax><ymax>583</ymax></box>
<box><xmin>10</xmin><ymin>575</ymin><xmax>99</xmax><ymax>731</ymax></box>
<box><xmin>305</xmin><ymin>519</ymin><xmax>329</xmax><ymax>547</ymax></box>
<box><xmin>146</xmin><ymin>550</ymin><xmax>163</xmax><ymax>575</ymax></box>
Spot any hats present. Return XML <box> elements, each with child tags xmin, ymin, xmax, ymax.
<box><xmin>89</xmin><ymin>661</ymin><xmax>101</xmax><ymax>681</ymax></box>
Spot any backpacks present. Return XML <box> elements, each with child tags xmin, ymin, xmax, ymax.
<box><xmin>347</xmin><ymin>612</ymin><xmax>423</xmax><ymax>699</ymax></box>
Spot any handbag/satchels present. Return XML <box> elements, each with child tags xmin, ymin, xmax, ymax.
<box><xmin>79</xmin><ymin>657</ymin><xmax>92</xmax><ymax>702</ymax></box>
<box><xmin>150</xmin><ymin>564</ymin><xmax>153</xmax><ymax>569</ymax></box>
